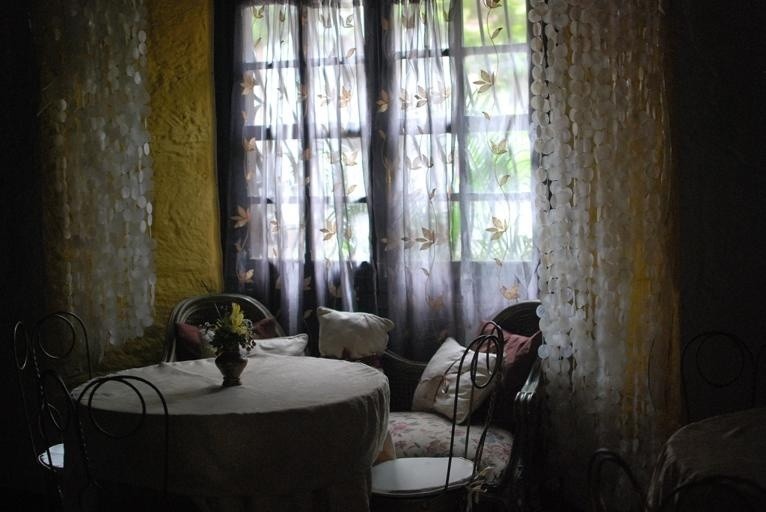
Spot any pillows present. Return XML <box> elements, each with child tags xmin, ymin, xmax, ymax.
<box><xmin>409</xmin><ymin>335</ymin><xmax>507</xmax><ymax>426</ymax></box>
<box><xmin>201</xmin><ymin>326</ymin><xmax>310</xmax><ymax>357</ymax></box>
<box><xmin>314</xmin><ymin>305</ymin><xmax>395</xmax><ymax>368</ymax></box>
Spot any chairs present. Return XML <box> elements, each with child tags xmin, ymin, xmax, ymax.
<box><xmin>588</xmin><ymin>407</ymin><xmax>766</xmax><ymax>509</ymax></box>
<box><xmin>11</xmin><ymin>311</ymin><xmax>94</xmax><ymax>512</ymax></box>
<box><xmin>63</xmin><ymin>373</ymin><xmax>171</xmax><ymax>512</ymax></box>
<box><xmin>374</xmin><ymin>320</ymin><xmax>506</xmax><ymax>512</ymax></box>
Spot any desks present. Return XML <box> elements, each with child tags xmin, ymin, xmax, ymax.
<box><xmin>72</xmin><ymin>355</ymin><xmax>389</xmax><ymax>512</ymax></box>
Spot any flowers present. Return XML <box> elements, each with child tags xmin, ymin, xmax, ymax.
<box><xmin>196</xmin><ymin>302</ymin><xmax>258</xmax><ymax>355</ymax></box>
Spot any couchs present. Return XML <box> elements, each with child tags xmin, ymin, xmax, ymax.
<box><xmin>165</xmin><ymin>293</ymin><xmax>544</xmax><ymax>488</ymax></box>
